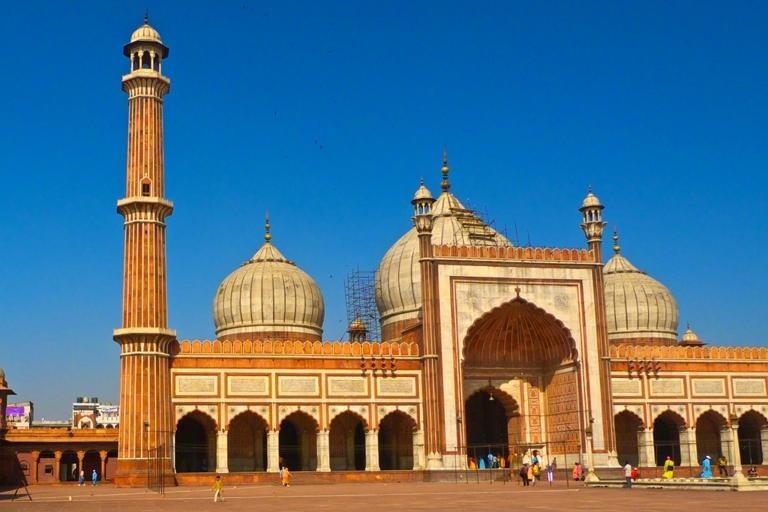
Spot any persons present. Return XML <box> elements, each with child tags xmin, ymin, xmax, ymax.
<box><xmin>281</xmin><ymin>468</ymin><xmax>293</xmax><ymax>488</ymax></box>
<box><xmin>90</xmin><ymin>470</ymin><xmax>98</xmax><ymax>487</ymax></box>
<box><xmin>469</xmin><ymin>454</ymin><xmax>506</xmax><ymax>470</ymax></box>
<box><xmin>76</xmin><ymin>471</ymin><xmax>86</xmax><ymax>487</ymax></box>
<box><xmin>717</xmin><ymin>456</ymin><xmax>729</xmax><ymax>476</ymax></box>
<box><xmin>699</xmin><ymin>455</ymin><xmax>713</xmax><ymax>477</ymax></box>
<box><xmin>519</xmin><ymin>449</ymin><xmax>554</xmax><ymax>488</ymax></box>
<box><xmin>623</xmin><ymin>461</ymin><xmax>632</xmax><ymax>489</ymax></box>
<box><xmin>572</xmin><ymin>462</ymin><xmax>582</xmax><ymax>482</ymax></box>
<box><xmin>211</xmin><ymin>476</ymin><xmax>225</xmax><ymax>503</ymax></box>
<box><xmin>747</xmin><ymin>465</ymin><xmax>758</xmax><ymax>477</ymax></box>
<box><xmin>662</xmin><ymin>455</ymin><xmax>675</xmax><ymax>479</ymax></box>
<box><xmin>630</xmin><ymin>467</ymin><xmax>638</xmax><ymax>483</ymax></box>
